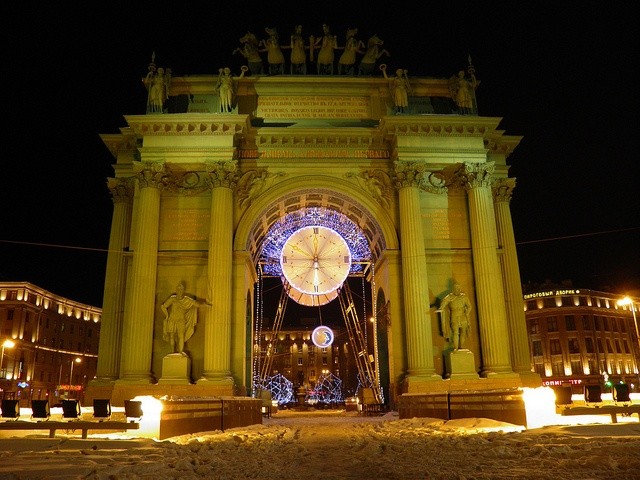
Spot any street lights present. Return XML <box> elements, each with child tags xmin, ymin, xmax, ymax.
<box><xmin>617</xmin><ymin>297</ymin><xmax>640</xmax><ymax>347</ymax></box>
<box><xmin>0</xmin><ymin>341</ymin><xmax>13</xmax><ymax>374</ymax></box>
<box><xmin>68</xmin><ymin>358</ymin><xmax>81</xmax><ymax>400</ymax></box>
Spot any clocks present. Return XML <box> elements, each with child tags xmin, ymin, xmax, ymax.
<box><xmin>280</xmin><ymin>227</ymin><xmax>352</xmax><ymax>295</ymax></box>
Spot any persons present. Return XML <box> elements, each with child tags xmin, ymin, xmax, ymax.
<box><xmin>216</xmin><ymin>66</ymin><xmax>244</xmax><ymax>111</ymax></box>
<box><xmin>439</xmin><ymin>283</ymin><xmax>473</xmax><ymax>351</ymax></box>
<box><xmin>143</xmin><ymin>67</ymin><xmax>169</xmax><ymax>113</ymax></box>
<box><xmin>161</xmin><ymin>283</ymin><xmax>214</xmax><ymax>354</ymax></box>
<box><xmin>454</xmin><ymin>71</ymin><xmax>477</xmax><ymax>114</ymax></box>
<box><xmin>382</xmin><ymin>66</ymin><xmax>410</xmax><ymax>114</ymax></box>
